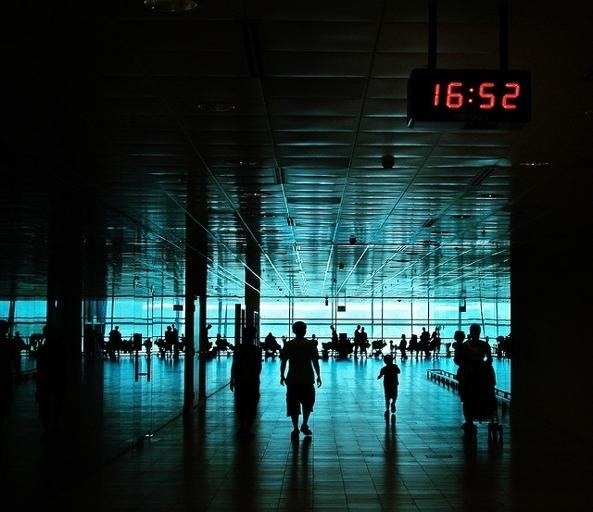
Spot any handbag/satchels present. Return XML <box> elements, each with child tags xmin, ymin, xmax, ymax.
<box><xmin>285</xmin><ymin>368</ymin><xmax>307</xmax><ymax>387</ymax></box>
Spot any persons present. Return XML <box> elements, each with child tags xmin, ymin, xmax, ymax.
<box><xmin>455</xmin><ymin>322</ymin><xmax>494</xmax><ymax>437</ymax></box>
<box><xmin>6</xmin><ymin>323</ymin><xmax>234</xmax><ymax>362</ymax></box>
<box><xmin>278</xmin><ymin>320</ymin><xmax>323</xmax><ymax>442</ymax></box>
<box><xmin>259</xmin><ymin>325</ymin><xmax>511</xmax><ymax>366</ymax></box>
<box><xmin>227</xmin><ymin>325</ymin><xmax>264</xmax><ymax>442</ymax></box>
<box><xmin>376</xmin><ymin>354</ymin><xmax>401</xmax><ymax>416</ymax></box>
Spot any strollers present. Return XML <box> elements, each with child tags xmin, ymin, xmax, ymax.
<box><xmin>458</xmin><ymin>362</ymin><xmax>503</xmax><ymax>432</ymax></box>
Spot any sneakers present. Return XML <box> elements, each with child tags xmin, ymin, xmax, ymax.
<box><xmin>290</xmin><ymin>425</ymin><xmax>312</xmax><ymax>445</ymax></box>
